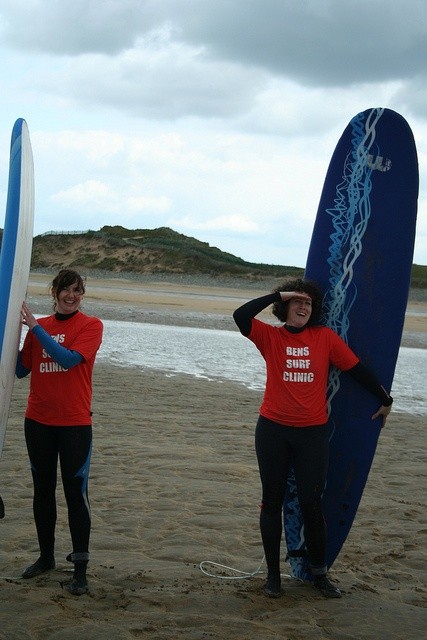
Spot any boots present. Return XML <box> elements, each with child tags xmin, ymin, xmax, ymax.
<box><xmin>263</xmin><ymin>534</ymin><xmax>282</xmax><ymax>596</ymax></box>
<box><xmin>67</xmin><ymin>552</ymin><xmax>88</xmax><ymax>594</ymax></box>
<box><xmin>22</xmin><ymin>537</ymin><xmax>55</xmax><ymax>578</ymax></box>
<box><xmin>312</xmin><ymin>563</ymin><xmax>340</xmax><ymax>598</ymax></box>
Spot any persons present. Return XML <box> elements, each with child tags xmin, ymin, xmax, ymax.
<box><xmin>14</xmin><ymin>270</ymin><xmax>104</xmax><ymax>596</ymax></box>
<box><xmin>233</xmin><ymin>280</ymin><xmax>393</xmax><ymax>599</ymax></box>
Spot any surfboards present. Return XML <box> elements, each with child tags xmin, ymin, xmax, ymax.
<box><xmin>0</xmin><ymin>116</ymin><xmax>35</xmax><ymax>460</ymax></box>
<box><xmin>283</xmin><ymin>107</ymin><xmax>418</xmax><ymax>585</ymax></box>
